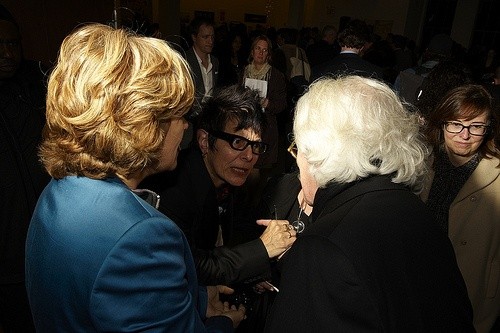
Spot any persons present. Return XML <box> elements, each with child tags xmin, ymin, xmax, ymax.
<box><xmin>0</xmin><ymin>8</ymin><xmax>500</xmax><ymax>180</ymax></box>
<box><xmin>261</xmin><ymin>73</ymin><xmax>476</xmax><ymax>332</ymax></box>
<box><xmin>24</xmin><ymin>24</ymin><xmax>246</xmax><ymax>332</ymax></box>
<box><xmin>145</xmin><ymin>87</ymin><xmax>297</xmax><ymax>287</ymax></box>
<box><xmin>422</xmin><ymin>85</ymin><xmax>500</xmax><ymax>333</ymax></box>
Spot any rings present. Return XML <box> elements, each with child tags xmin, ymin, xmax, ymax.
<box><xmin>284</xmin><ymin>224</ymin><xmax>293</xmax><ymax>238</ymax></box>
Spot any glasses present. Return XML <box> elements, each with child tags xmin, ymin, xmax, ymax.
<box><xmin>211</xmin><ymin>130</ymin><xmax>271</xmax><ymax>156</ymax></box>
<box><xmin>441</xmin><ymin>120</ymin><xmax>490</xmax><ymax>137</ymax></box>
<box><xmin>286</xmin><ymin>138</ymin><xmax>299</xmax><ymax>160</ymax></box>
